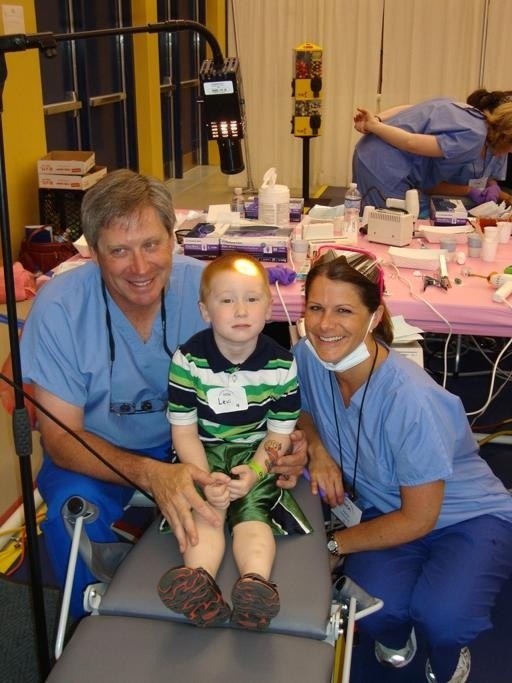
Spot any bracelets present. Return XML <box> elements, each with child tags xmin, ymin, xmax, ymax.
<box><xmin>374</xmin><ymin>115</ymin><xmax>384</xmax><ymax>122</ymax></box>
<box><xmin>250</xmin><ymin>463</ymin><xmax>266</xmax><ymax>485</ymax></box>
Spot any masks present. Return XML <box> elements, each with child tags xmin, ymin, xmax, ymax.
<box><xmin>301</xmin><ymin>313</ymin><xmax>375</xmax><ymax>372</ymax></box>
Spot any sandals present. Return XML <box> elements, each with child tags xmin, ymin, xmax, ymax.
<box><xmin>228</xmin><ymin>571</ymin><xmax>281</xmax><ymax>632</ymax></box>
<box><xmin>157</xmin><ymin>564</ymin><xmax>232</xmax><ymax>629</ymax></box>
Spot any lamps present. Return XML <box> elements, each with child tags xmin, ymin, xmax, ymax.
<box><xmin>199</xmin><ymin>56</ymin><xmax>246</xmax><ymax>174</ymax></box>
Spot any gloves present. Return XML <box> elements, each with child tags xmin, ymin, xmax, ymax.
<box><xmin>469</xmin><ymin>186</ymin><xmax>485</xmax><ymax>204</ymax></box>
<box><xmin>481</xmin><ymin>185</ymin><xmax>500</xmax><ymax>204</ymax></box>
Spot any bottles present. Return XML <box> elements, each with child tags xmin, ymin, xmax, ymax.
<box><xmin>344</xmin><ymin>182</ymin><xmax>362</xmax><ymax>222</ymax></box>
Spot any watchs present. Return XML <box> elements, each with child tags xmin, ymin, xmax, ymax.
<box><xmin>327</xmin><ymin>530</ymin><xmax>343</xmax><ymax>559</ymax></box>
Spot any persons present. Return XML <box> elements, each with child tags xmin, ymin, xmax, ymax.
<box><xmin>346</xmin><ymin>97</ymin><xmax>512</xmax><ymax>210</ymax></box>
<box><xmin>19</xmin><ymin>171</ymin><xmax>210</xmax><ymax>625</ymax></box>
<box><xmin>157</xmin><ymin>250</ymin><xmax>300</xmax><ymax>631</ymax></box>
<box><xmin>292</xmin><ymin>253</ymin><xmax>511</xmax><ymax>682</ymax></box>
<box><xmin>416</xmin><ymin>89</ymin><xmax>507</xmax><ymax>215</ymax></box>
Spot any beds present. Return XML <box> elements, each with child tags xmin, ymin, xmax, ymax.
<box><xmin>45</xmin><ymin>469</ymin><xmax>362</xmax><ymax>683</ymax></box>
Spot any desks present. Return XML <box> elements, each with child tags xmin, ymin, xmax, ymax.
<box><xmin>35</xmin><ymin>207</ymin><xmax>511</xmax><ymax>337</ymax></box>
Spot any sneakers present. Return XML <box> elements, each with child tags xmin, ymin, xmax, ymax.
<box><xmin>374</xmin><ymin>627</ymin><xmax>417</xmax><ymax>669</ymax></box>
<box><xmin>424</xmin><ymin>646</ymin><xmax>470</xmax><ymax>683</ymax></box>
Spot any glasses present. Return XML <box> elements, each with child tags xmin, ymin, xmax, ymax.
<box><xmin>109</xmin><ymin>358</ymin><xmax>167</xmax><ymax>415</ymax></box>
<box><xmin>310</xmin><ymin>245</ymin><xmax>384</xmax><ymax>306</ymax></box>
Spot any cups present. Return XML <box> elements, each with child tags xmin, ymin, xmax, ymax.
<box><xmin>498</xmin><ymin>222</ymin><xmax>512</xmax><ymax>243</ymax></box>
<box><xmin>483</xmin><ymin>240</ymin><xmax>497</xmax><ymax>261</ymax></box>
<box><xmin>485</xmin><ymin>226</ymin><xmax>498</xmax><ymax>238</ymax></box>
